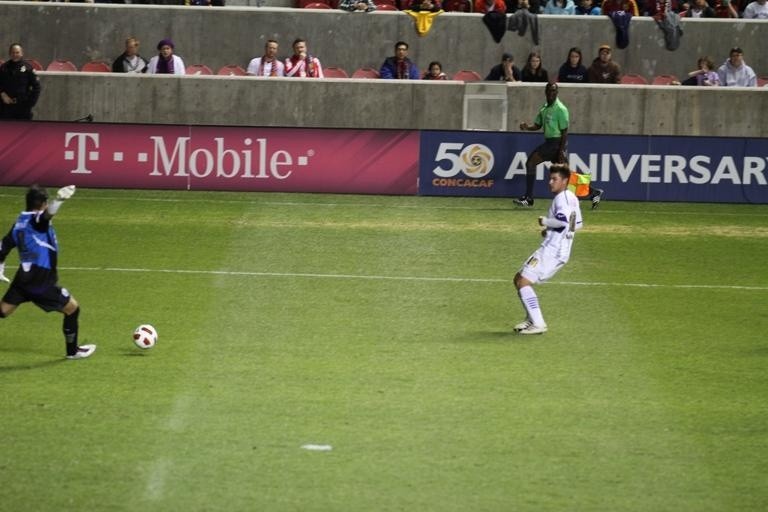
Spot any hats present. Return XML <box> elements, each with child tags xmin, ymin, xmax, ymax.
<box><xmin>600</xmin><ymin>44</ymin><xmax>611</xmax><ymax>50</ymax></box>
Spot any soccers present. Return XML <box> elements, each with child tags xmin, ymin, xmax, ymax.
<box><xmin>133</xmin><ymin>324</ymin><xmax>156</xmax><ymax>350</ymax></box>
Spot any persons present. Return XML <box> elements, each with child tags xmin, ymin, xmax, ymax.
<box><xmin>144</xmin><ymin>39</ymin><xmax>188</xmax><ymax>77</ymax></box>
<box><xmin>1</xmin><ymin>43</ymin><xmax>41</xmax><ymax>121</ymax></box>
<box><xmin>483</xmin><ymin>45</ymin><xmax>621</xmax><ymax>83</ymax></box>
<box><xmin>512</xmin><ymin>84</ymin><xmax>606</xmax><ymax>209</ymax></box>
<box><xmin>285</xmin><ymin>39</ymin><xmax>329</xmax><ymax>78</ymax></box>
<box><xmin>379</xmin><ymin>41</ymin><xmax>419</xmax><ymax>80</ymax></box>
<box><xmin>243</xmin><ymin>38</ymin><xmax>284</xmax><ymax>76</ymax></box>
<box><xmin>512</xmin><ymin>164</ymin><xmax>584</xmax><ymax>336</ymax></box>
<box><xmin>111</xmin><ymin>37</ymin><xmax>148</xmax><ymax>77</ymax></box>
<box><xmin>715</xmin><ymin>46</ymin><xmax>758</xmax><ymax>86</ymax></box>
<box><xmin>423</xmin><ymin>62</ymin><xmax>451</xmax><ymax>80</ymax></box>
<box><xmin>341</xmin><ymin>0</ymin><xmax>376</xmax><ymax>13</ymax></box>
<box><xmin>687</xmin><ymin>56</ymin><xmax>722</xmax><ymax>86</ymax></box>
<box><xmin>0</xmin><ymin>184</ymin><xmax>97</xmax><ymax>361</ymax></box>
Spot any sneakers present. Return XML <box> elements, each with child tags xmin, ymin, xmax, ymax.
<box><xmin>591</xmin><ymin>188</ymin><xmax>604</xmax><ymax>209</ymax></box>
<box><xmin>65</xmin><ymin>344</ymin><xmax>96</xmax><ymax>359</ymax></box>
<box><xmin>512</xmin><ymin>194</ymin><xmax>533</xmax><ymax>207</ymax></box>
<box><xmin>519</xmin><ymin>322</ymin><xmax>549</xmax><ymax>335</ymax></box>
<box><xmin>513</xmin><ymin>319</ymin><xmax>533</xmax><ymax>331</ymax></box>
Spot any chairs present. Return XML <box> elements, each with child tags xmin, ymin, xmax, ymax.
<box><xmin>25</xmin><ymin>57</ymin><xmax>766</xmax><ymax>88</ymax></box>
<box><xmin>301</xmin><ymin>2</ymin><xmax>741</xmax><ymax>18</ymax></box>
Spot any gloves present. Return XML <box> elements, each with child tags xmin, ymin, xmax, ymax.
<box><xmin>58</xmin><ymin>185</ymin><xmax>75</xmax><ymax>198</ymax></box>
